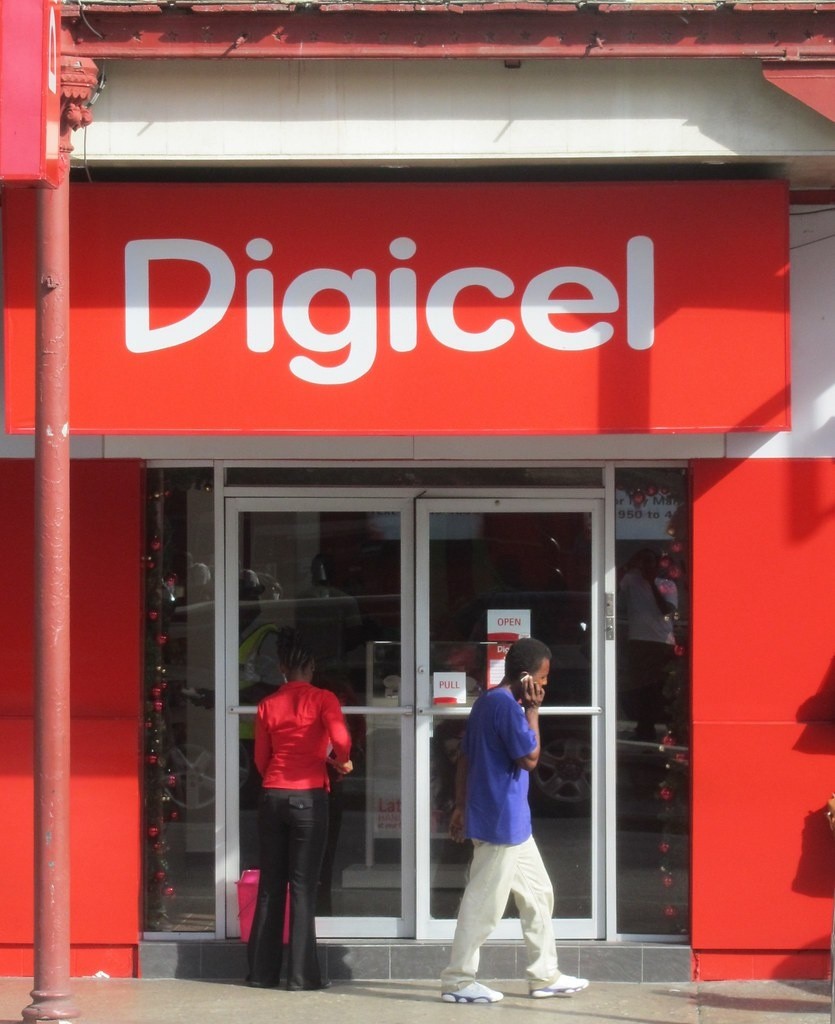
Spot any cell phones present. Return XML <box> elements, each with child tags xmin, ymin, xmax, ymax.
<box><xmin>521</xmin><ymin>675</ymin><xmax>530</xmax><ymax>683</ymax></box>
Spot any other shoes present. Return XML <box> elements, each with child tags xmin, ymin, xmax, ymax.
<box><xmin>321</xmin><ymin>979</ymin><xmax>332</xmax><ymax>988</ymax></box>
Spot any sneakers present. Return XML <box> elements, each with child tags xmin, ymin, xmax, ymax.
<box><xmin>442</xmin><ymin>981</ymin><xmax>503</xmax><ymax>1005</ymax></box>
<box><xmin>530</xmin><ymin>974</ymin><xmax>589</xmax><ymax>998</ymax></box>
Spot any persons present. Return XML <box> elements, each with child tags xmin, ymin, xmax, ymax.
<box><xmin>244</xmin><ymin>640</ymin><xmax>353</xmax><ymax>990</ymax></box>
<box><xmin>619</xmin><ymin>545</ymin><xmax>679</xmax><ymax>739</ymax></box>
<box><xmin>441</xmin><ymin>638</ymin><xmax>589</xmax><ymax>1004</ymax></box>
